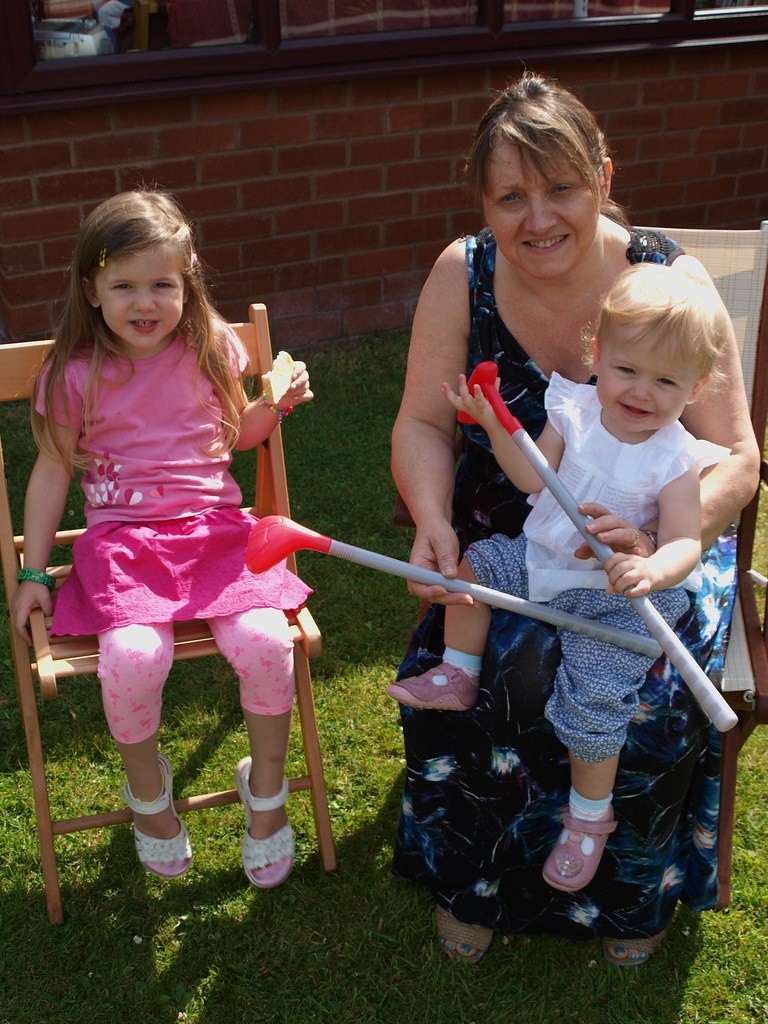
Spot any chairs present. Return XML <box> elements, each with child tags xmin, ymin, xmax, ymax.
<box><xmin>406</xmin><ymin>218</ymin><xmax>767</xmax><ymax>912</ymax></box>
<box><xmin>1</xmin><ymin>305</ymin><xmax>337</xmax><ymax>922</ymax></box>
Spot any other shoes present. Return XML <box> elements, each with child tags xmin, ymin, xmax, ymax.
<box><xmin>543</xmin><ymin>804</ymin><xmax>618</xmax><ymax>892</ymax></box>
<box><xmin>387</xmin><ymin>663</ymin><xmax>480</xmax><ymax>711</ymax></box>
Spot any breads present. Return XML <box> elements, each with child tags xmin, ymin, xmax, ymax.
<box><xmin>261</xmin><ymin>351</ymin><xmax>296</xmax><ymax>405</ymax></box>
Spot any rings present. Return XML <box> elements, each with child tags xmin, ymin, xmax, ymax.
<box><xmin>629</xmin><ymin>529</ymin><xmax>639</xmax><ymax>546</ymax></box>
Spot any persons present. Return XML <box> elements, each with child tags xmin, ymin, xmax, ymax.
<box><xmin>11</xmin><ymin>189</ymin><xmax>314</xmax><ymax>888</ymax></box>
<box><xmin>388</xmin><ymin>262</ymin><xmax>731</xmax><ymax>892</ymax></box>
<box><xmin>390</xmin><ymin>76</ymin><xmax>760</xmax><ymax>965</ymax></box>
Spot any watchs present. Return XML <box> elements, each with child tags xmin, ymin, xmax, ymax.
<box><xmin>642</xmin><ymin>530</ymin><xmax>657</xmax><ymax>547</ymax></box>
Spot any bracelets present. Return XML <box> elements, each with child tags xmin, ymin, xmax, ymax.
<box><xmin>263</xmin><ymin>390</ymin><xmax>292</xmax><ymax>425</ymax></box>
<box><xmin>16</xmin><ymin>568</ymin><xmax>56</xmax><ymax>591</ymax></box>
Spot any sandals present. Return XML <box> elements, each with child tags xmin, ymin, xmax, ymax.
<box><xmin>236</xmin><ymin>756</ymin><xmax>295</xmax><ymax>889</ymax></box>
<box><xmin>602</xmin><ymin>929</ymin><xmax>667</xmax><ymax>966</ymax></box>
<box><xmin>435</xmin><ymin>904</ymin><xmax>493</xmax><ymax>963</ymax></box>
<box><xmin>125</xmin><ymin>754</ymin><xmax>191</xmax><ymax>878</ymax></box>
